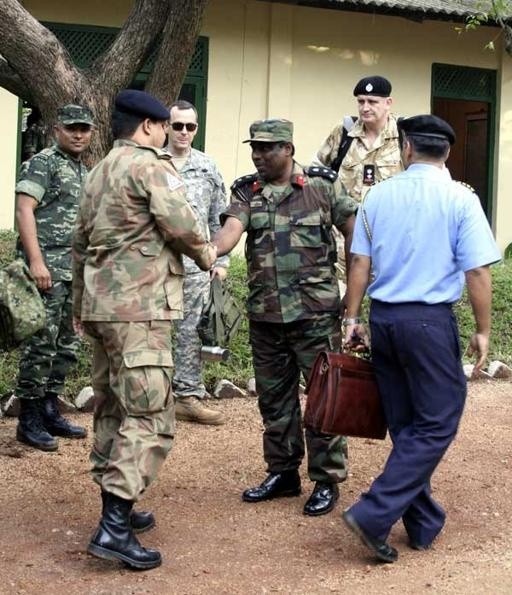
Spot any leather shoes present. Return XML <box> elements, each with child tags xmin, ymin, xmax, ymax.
<box><xmin>303</xmin><ymin>479</ymin><xmax>340</xmax><ymax>515</ymax></box>
<box><xmin>240</xmin><ymin>469</ymin><xmax>302</xmax><ymax>503</ymax></box>
<box><xmin>175</xmin><ymin>396</ymin><xmax>224</xmax><ymax>425</ymax></box>
<box><xmin>341</xmin><ymin>509</ymin><xmax>399</xmax><ymax>561</ymax></box>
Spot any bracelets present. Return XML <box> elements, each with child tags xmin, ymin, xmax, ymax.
<box><xmin>342</xmin><ymin>317</ymin><xmax>362</xmax><ymax>326</ymax></box>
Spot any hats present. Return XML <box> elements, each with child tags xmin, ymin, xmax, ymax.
<box><xmin>55</xmin><ymin>104</ymin><xmax>95</xmax><ymax>127</ymax></box>
<box><xmin>397</xmin><ymin>114</ymin><xmax>457</xmax><ymax>144</ymax></box>
<box><xmin>354</xmin><ymin>76</ymin><xmax>393</xmax><ymax>98</ymax></box>
<box><xmin>115</xmin><ymin>89</ymin><xmax>169</xmax><ymax>119</ymax></box>
<box><xmin>242</xmin><ymin>119</ymin><xmax>295</xmax><ymax>146</ymax></box>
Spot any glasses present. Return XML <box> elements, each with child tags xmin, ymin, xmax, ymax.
<box><xmin>167</xmin><ymin>122</ymin><xmax>198</xmax><ymax>132</ymax></box>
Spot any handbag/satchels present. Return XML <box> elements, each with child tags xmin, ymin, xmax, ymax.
<box><xmin>302</xmin><ymin>342</ymin><xmax>390</xmax><ymax>441</ymax></box>
<box><xmin>196</xmin><ymin>271</ymin><xmax>244</xmax><ymax>349</ymax></box>
<box><xmin>0</xmin><ymin>258</ymin><xmax>48</xmax><ymax>350</ymax></box>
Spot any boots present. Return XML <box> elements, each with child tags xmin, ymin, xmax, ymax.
<box><xmin>86</xmin><ymin>490</ymin><xmax>162</xmax><ymax>569</ymax></box>
<box><xmin>16</xmin><ymin>389</ymin><xmax>86</xmax><ymax>452</ymax></box>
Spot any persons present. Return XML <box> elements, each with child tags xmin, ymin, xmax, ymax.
<box><xmin>164</xmin><ymin>98</ymin><xmax>225</xmax><ymax>425</ymax></box>
<box><xmin>339</xmin><ymin>113</ymin><xmax>502</xmax><ymax>563</ymax></box>
<box><xmin>22</xmin><ymin>109</ymin><xmax>46</xmax><ymax>157</ymax></box>
<box><xmin>13</xmin><ymin>104</ymin><xmax>88</xmax><ymax>451</ymax></box>
<box><xmin>72</xmin><ymin>88</ymin><xmax>218</xmax><ymax>573</ymax></box>
<box><xmin>312</xmin><ymin>73</ymin><xmax>406</xmax><ymax>295</ymax></box>
<box><xmin>213</xmin><ymin>117</ymin><xmax>360</xmax><ymax>515</ymax></box>
